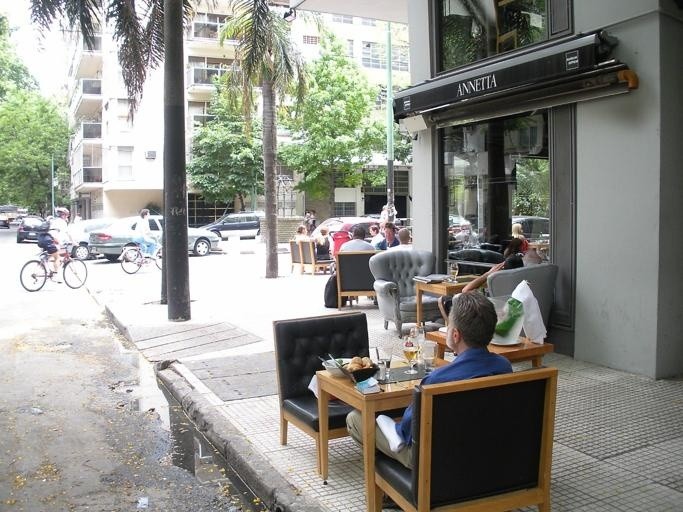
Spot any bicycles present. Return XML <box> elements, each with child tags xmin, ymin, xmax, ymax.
<box><xmin>116</xmin><ymin>244</ymin><xmax>162</xmax><ymax>274</ymax></box>
<box><xmin>20</xmin><ymin>241</ymin><xmax>88</xmax><ymax>292</ymax></box>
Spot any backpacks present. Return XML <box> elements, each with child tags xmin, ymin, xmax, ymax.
<box><xmin>38</xmin><ymin>217</ymin><xmax>53</xmax><ymax>234</ymax></box>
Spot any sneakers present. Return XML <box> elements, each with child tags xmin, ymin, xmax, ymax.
<box><xmin>52</xmin><ymin>272</ymin><xmax>62</xmax><ymax>283</ymax></box>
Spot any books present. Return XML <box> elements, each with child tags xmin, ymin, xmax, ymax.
<box><xmin>355</xmin><ymin>377</ymin><xmax>380</xmax><ymax>394</ymax></box>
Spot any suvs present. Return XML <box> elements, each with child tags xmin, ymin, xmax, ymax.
<box><xmin>201</xmin><ymin>213</ymin><xmax>260</xmax><ymax>240</ymax></box>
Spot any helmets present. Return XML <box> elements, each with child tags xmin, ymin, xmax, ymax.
<box><xmin>54</xmin><ymin>207</ymin><xmax>69</xmax><ymax>217</ymax></box>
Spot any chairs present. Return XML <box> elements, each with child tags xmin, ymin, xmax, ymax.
<box><xmin>374</xmin><ymin>366</ymin><xmax>558</xmax><ymax>512</ymax></box>
<box><xmin>300</xmin><ymin>241</ymin><xmax>335</xmax><ymax>277</ymax></box>
<box><xmin>289</xmin><ymin>238</ymin><xmax>303</xmax><ymax>275</ymax></box>
<box><xmin>336</xmin><ymin>250</ymin><xmax>385</xmax><ymax>311</ymax></box>
<box><xmin>273</xmin><ymin>311</ymin><xmax>370</xmax><ymax>477</ymax></box>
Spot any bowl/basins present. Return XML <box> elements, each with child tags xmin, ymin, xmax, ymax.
<box><xmin>322</xmin><ymin>358</ymin><xmax>354</xmax><ymax>377</ymax></box>
<box><xmin>341</xmin><ymin>362</ymin><xmax>379</xmax><ymax>382</ymax></box>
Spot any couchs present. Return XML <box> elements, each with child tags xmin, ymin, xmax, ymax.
<box><xmin>369</xmin><ymin>248</ymin><xmax>446</xmax><ymax>339</ymax></box>
<box><xmin>449</xmin><ymin>249</ymin><xmax>506</xmax><ymax>275</ymax></box>
<box><xmin>454</xmin><ymin>262</ymin><xmax>559</xmax><ymax>356</ymax></box>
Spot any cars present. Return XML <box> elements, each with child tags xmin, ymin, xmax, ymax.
<box><xmin>512</xmin><ymin>216</ymin><xmax>549</xmax><ymax>239</ymax></box>
<box><xmin>16</xmin><ymin>216</ymin><xmax>44</xmax><ymax>243</ymax></box>
<box><xmin>0</xmin><ymin>216</ymin><xmax>10</xmax><ymax>229</ymax></box>
<box><xmin>62</xmin><ymin>217</ymin><xmax>114</xmax><ymax>260</ymax></box>
<box><xmin>88</xmin><ymin>214</ymin><xmax>219</xmax><ymax>261</ymax></box>
<box><xmin>310</xmin><ymin>216</ymin><xmax>380</xmax><ymax>240</ymax></box>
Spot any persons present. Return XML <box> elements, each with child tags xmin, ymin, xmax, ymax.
<box><xmin>294</xmin><ymin>225</ymin><xmax>311</xmax><ymax>244</ymax></box>
<box><xmin>369</xmin><ymin>224</ymin><xmax>384</xmax><ymax>245</ymax></box>
<box><xmin>448</xmin><ymin>235</ymin><xmax>461</xmax><ymax>250</ymax></box>
<box><xmin>316</xmin><ymin>227</ymin><xmax>330</xmax><ymax>260</ymax></box>
<box><xmin>511</xmin><ymin>222</ymin><xmax>529</xmax><ymax>251</ymax></box>
<box><xmin>438</xmin><ymin>255</ymin><xmax>524</xmax><ymax>325</ymax></box>
<box><xmin>38</xmin><ymin>207</ymin><xmax>69</xmax><ymax>284</ymax></box>
<box><xmin>374</xmin><ymin>222</ymin><xmax>399</xmax><ymax>249</ymax></box>
<box><xmin>132</xmin><ymin>209</ymin><xmax>159</xmax><ymax>261</ymax></box>
<box><xmin>345</xmin><ymin>292</ymin><xmax>513</xmax><ymax>470</ymax></box>
<box><xmin>504</xmin><ymin>238</ymin><xmax>523</xmax><ymax>256</ymax></box>
<box><xmin>339</xmin><ymin>226</ymin><xmax>375</xmax><ymax>250</ymax></box>
<box><xmin>455</xmin><ymin>222</ymin><xmax>476</xmax><ymax>242</ymax></box>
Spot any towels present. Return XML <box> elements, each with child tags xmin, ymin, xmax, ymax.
<box><xmin>376</xmin><ymin>414</ymin><xmax>404</xmax><ymax>453</ymax></box>
<box><xmin>511</xmin><ymin>279</ymin><xmax>546</xmax><ymax>345</ymax></box>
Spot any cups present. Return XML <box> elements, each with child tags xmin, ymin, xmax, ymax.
<box><xmin>421</xmin><ymin>342</ymin><xmax>438</xmax><ymax>371</ymax></box>
<box><xmin>450</xmin><ymin>264</ymin><xmax>459</xmax><ymax>281</ymax></box>
<box><xmin>376</xmin><ymin>346</ymin><xmax>393</xmax><ymax>379</ymax></box>
<box><xmin>402</xmin><ymin>340</ymin><xmax>420</xmax><ymax>374</ymax></box>
